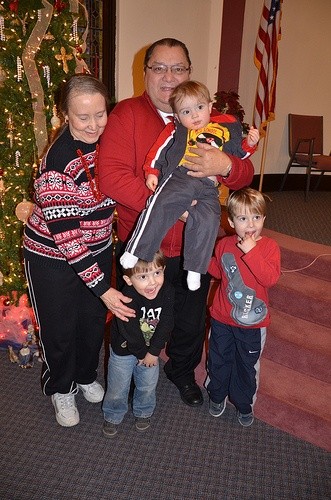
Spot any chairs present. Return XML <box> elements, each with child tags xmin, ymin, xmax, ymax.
<box><xmin>279</xmin><ymin>113</ymin><xmax>331</xmax><ymax>202</ymax></box>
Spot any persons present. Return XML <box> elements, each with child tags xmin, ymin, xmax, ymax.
<box><xmin>23</xmin><ymin>74</ymin><xmax>137</xmax><ymax>428</ymax></box>
<box><xmin>119</xmin><ymin>79</ymin><xmax>260</xmax><ymax>290</ymax></box>
<box><xmin>95</xmin><ymin>35</ymin><xmax>255</xmax><ymax>406</ymax></box>
<box><xmin>101</xmin><ymin>246</ymin><xmax>174</xmax><ymax>437</ymax></box>
<box><xmin>204</xmin><ymin>187</ymin><xmax>282</xmax><ymax>426</ymax></box>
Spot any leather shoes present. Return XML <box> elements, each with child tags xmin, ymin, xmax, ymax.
<box><xmin>179</xmin><ymin>374</ymin><xmax>204</xmax><ymax>406</ymax></box>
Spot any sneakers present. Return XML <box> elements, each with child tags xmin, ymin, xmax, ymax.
<box><xmin>135</xmin><ymin>417</ymin><xmax>151</xmax><ymax>430</ymax></box>
<box><xmin>209</xmin><ymin>397</ymin><xmax>227</xmax><ymax>417</ymax></box>
<box><xmin>103</xmin><ymin>420</ymin><xmax>119</xmax><ymax>435</ymax></box>
<box><xmin>50</xmin><ymin>392</ymin><xmax>80</xmax><ymax>428</ymax></box>
<box><xmin>237</xmin><ymin>408</ymin><xmax>255</xmax><ymax>427</ymax></box>
<box><xmin>76</xmin><ymin>373</ymin><xmax>105</xmax><ymax>403</ymax></box>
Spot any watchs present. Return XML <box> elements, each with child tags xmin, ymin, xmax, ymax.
<box><xmin>227</xmin><ymin>166</ymin><xmax>232</xmax><ymax>175</ymax></box>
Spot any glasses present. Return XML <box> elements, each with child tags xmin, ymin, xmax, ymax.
<box><xmin>143</xmin><ymin>62</ymin><xmax>190</xmax><ymax>74</ymax></box>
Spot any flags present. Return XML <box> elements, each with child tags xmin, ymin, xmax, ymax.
<box><xmin>252</xmin><ymin>0</ymin><xmax>284</xmax><ymax>150</ymax></box>
